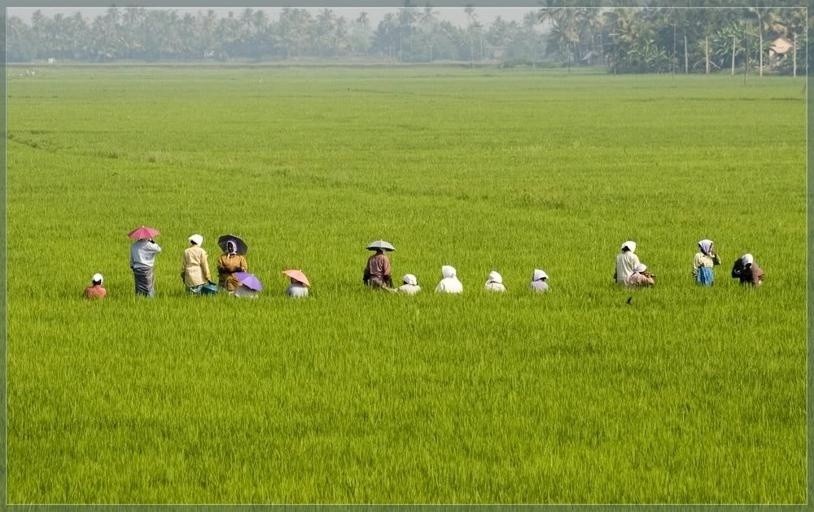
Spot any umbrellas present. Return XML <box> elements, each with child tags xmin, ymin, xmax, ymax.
<box><xmin>282</xmin><ymin>269</ymin><xmax>311</xmax><ymax>289</ymax></box>
<box><xmin>128</xmin><ymin>226</ymin><xmax>160</xmax><ymax>240</ymax></box>
<box><xmin>217</xmin><ymin>235</ymin><xmax>248</xmax><ymax>255</ymax></box>
<box><xmin>367</xmin><ymin>239</ymin><xmax>396</xmax><ymax>252</ymax></box>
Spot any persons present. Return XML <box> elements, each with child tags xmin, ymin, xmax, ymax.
<box><xmin>617</xmin><ymin>240</ymin><xmax>641</xmax><ymax>283</ymax></box>
<box><xmin>692</xmin><ymin>239</ymin><xmax>721</xmax><ymax>287</ymax></box>
<box><xmin>530</xmin><ymin>268</ymin><xmax>549</xmax><ymax>292</ymax></box>
<box><xmin>485</xmin><ymin>271</ymin><xmax>507</xmax><ymax>292</ymax></box>
<box><xmin>382</xmin><ymin>274</ymin><xmax>421</xmax><ymax>296</ymax></box>
<box><xmin>181</xmin><ymin>235</ymin><xmax>211</xmax><ymax>294</ymax></box>
<box><xmin>216</xmin><ymin>240</ymin><xmax>259</xmax><ymax>299</ymax></box>
<box><xmin>363</xmin><ymin>248</ymin><xmax>394</xmax><ymax>291</ymax></box>
<box><xmin>732</xmin><ymin>253</ymin><xmax>765</xmax><ymax>287</ymax></box>
<box><xmin>629</xmin><ymin>264</ymin><xmax>657</xmax><ymax>287</ymax></box>
<box><xmin>130</xmin><ymin>237</ymin><xmax>161</xmax><ymax>297</ymax></box>
<box><xmin>286</xmin><ymin>277</ymin><xmax>308</xmax><ymax>298</ymax></box>
<box><xmin>434</xmin><ymin>265</ymin><xmax>463</xmax><ymax>294</ymax></box>
<box><xmin>84</xmin><ymin>273</ymin><xmax>107</xmax><ymax>300</ymax></box>
